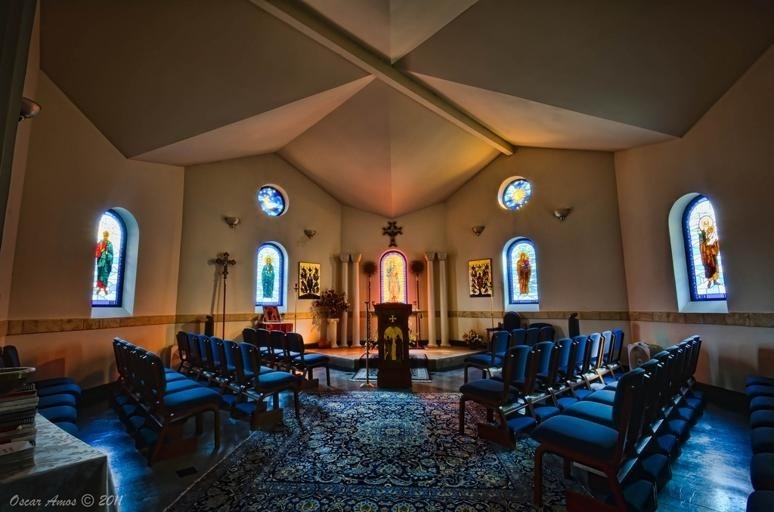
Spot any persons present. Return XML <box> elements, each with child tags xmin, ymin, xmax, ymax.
<box><xmin>385</xmin><ymin>261</ymin><xmax>401</xmax><ymax>299</ymax></box>
<box><xmin>516</xmin><ymin>253</ymin><xmax>529</xmax><ymax>294</ymax></box>
<box><xmin>262</xmin><ymin>257</ymin><xmax>274</xmax><ymax>298</ymax></box>
<box><xmin>95</xmin><ymin>230</ymin><xmax>113</xmax><ymax>295</ymax></box>
<box><xmin>699</xmin><ymin>230</ymin><xmax>721</xmax><ymax>289</ymax></box>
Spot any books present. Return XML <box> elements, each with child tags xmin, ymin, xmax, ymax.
<box><xmin>0</xmin><ymin>383</ymin><xmax>41</xmax><ymax>478</ymax></box>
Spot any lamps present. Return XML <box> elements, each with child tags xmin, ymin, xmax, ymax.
<box><xmin>226</xmin><ymin>216</ymin><xmax>240</xmax><ymax>229</ymax></box>
<box><xmin>303</xmin><ymin>228</ymin><xmax>319</xmax><ymax>239</ymax></box>
<box><xmin>472</xmin><ymin>224</ymin><xmax>486</xmax><ymax>236</ymax></box>
<box><xmin>552</xmin><ymin>207</ymin><xmax>571</xmax><ymax>224</ymax></box>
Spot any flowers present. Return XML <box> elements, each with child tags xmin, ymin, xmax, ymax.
<box><xmin>311</xmin><ymin>288</ymin><xmax>352</xmax><ymax>319</ymax></box>
<box><xmin>462</xmin><ymin>328</ymin><xmax>484</xmax><ymax>349</ymax></box>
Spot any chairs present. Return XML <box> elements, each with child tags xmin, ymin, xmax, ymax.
<box><xmin>1</xmin><ymin>324</ymin><xmax>332</xmax><ymax>511</ymax></box>
<box><xmin>458</xmin><ymin>323</ymin><xmax>774</xmax><ymax>511</ymax></box>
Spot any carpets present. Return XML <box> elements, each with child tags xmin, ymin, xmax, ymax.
<box><xmin>164</xmin><ymin>387</ymin><xmax>605</xmax><ymax>510</ymax></box>
<box><xmin>347</xmin><ymin>351</ymin><xmax>433</xmax><ymax>384</ymax></box>
